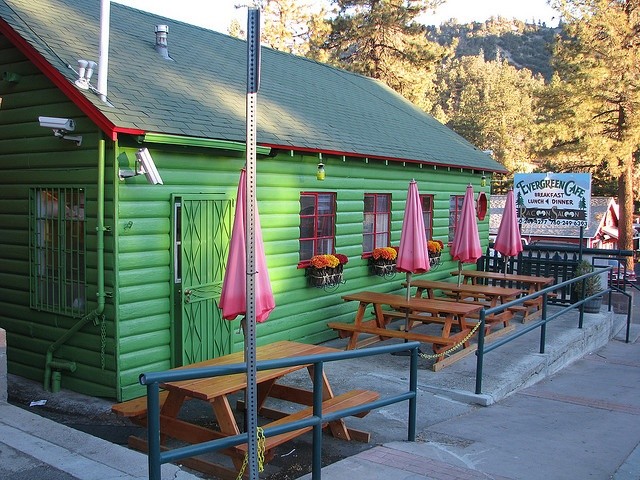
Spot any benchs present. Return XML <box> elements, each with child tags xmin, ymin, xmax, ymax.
<box><xmin>520</xmin><ymin>290</ymin><xmax>558</xmax><ymax>297</ymax></box>
<box><xmin>112</xmin><ymin>388</ymin><xmax>193</xmax><ymax>418</ymax></box>
<box><xmin>442</xmin><ymin>291</ymin><xmax>540</xmax><ymax>306</ymax></box>
<box><xmin>371</xmin><ymin>309</ymin><xmax>479</xmax><ymax>329</ymax></box>
<box><xmin>390</xmin><ymin>305</ymin><xmax>494</xmax><ymax>320</ymax></box>
<box><xmin>422</xmin><ymin>297</ymin><xmax>528</xmax><ymax>312</ymax></box>
<box><xmin>231</xmin><ymin>389</ymin><xmax>380</xmax><ymax>457</ymax></box>
<box><xmin>327</xmin><ymin>322</ymin><xmax>460</xmax><ymax>347</ymax></box>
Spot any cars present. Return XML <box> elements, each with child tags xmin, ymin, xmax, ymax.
<box><xmin>608</xmin><ymin>260</ymin><xmax>637</xmax><ymax>289</ymax></box>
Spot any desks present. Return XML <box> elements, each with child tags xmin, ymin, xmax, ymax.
<box><xmin>339</xmin><ymin>290</ymin><xmax>484</xmax><ymax>373</ymax></box>
<box><xmin>450</xmin><ymin>270</ymin><xmax>556</xmax><ymax>324</ymax></box>
<box><xmin>399</xmin><ymin>280</ymin><xmax>523</xmax><ymax>344</ymax></box>
<box><xmin>132</xmin><ymin>340</ymin><xmax>371</xmax><ymax>479</ymax></box>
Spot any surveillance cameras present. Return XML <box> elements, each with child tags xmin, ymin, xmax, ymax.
<box><xmin>39</xmin><ymin>115</ymin><xmax>82</xmax><ymax>147</ymax></box>
<box><xmin>118</xmin><ymin>148</ymin><xmax>165</xmax><ymax>186</ymax></box>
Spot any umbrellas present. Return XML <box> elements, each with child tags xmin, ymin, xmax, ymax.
<box><xmin>442</xmin><ymin>184</ymin><xmax>483</xmax><ymax>332</ymax></box>
<box><xmin>392</xmin><ymin>178</ymin><xmax>431</xmax><ymax>356</ymax></box>
<box><xmin>494</xmin><ymin>186</ymin><xmax>523</xmax><ymax>275</ymax></box>
<box><xmin>219</xmin><ymin>165</ymin><xmax>277</xmax><ymax>434</ymax></box>
